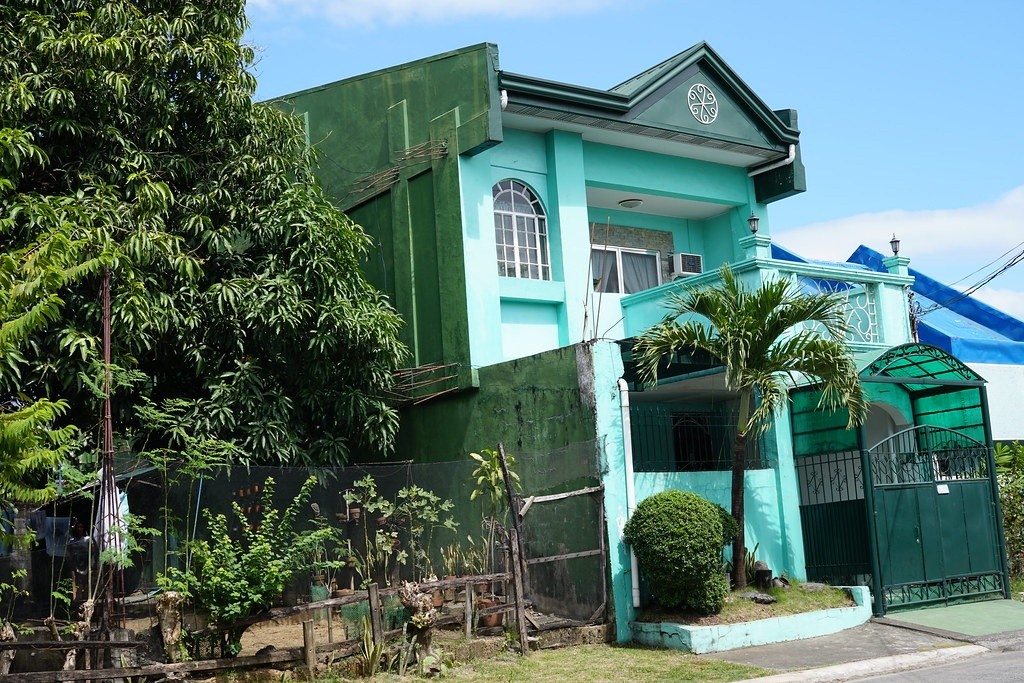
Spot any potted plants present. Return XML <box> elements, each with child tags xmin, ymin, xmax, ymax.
<box><xmin>278</xmin><ymin>447</ymin><xmax>524</xmax><ymax>629</ymax></box>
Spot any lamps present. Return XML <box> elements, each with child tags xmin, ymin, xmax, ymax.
<box><xmin>746</xmin><ymin>209</ymin><xmax>760</xmax><ymax>234</ymax></box>
<box><xmin>889</xmin><ymin>232</ymin><xmax>901</xmax><ymax>254</ymax></box>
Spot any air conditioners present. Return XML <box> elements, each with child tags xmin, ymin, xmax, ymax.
<box><xmin>673</xmin><ymin>252</ymin><xmax>704</xmax><ymax>276</ymax></box>
